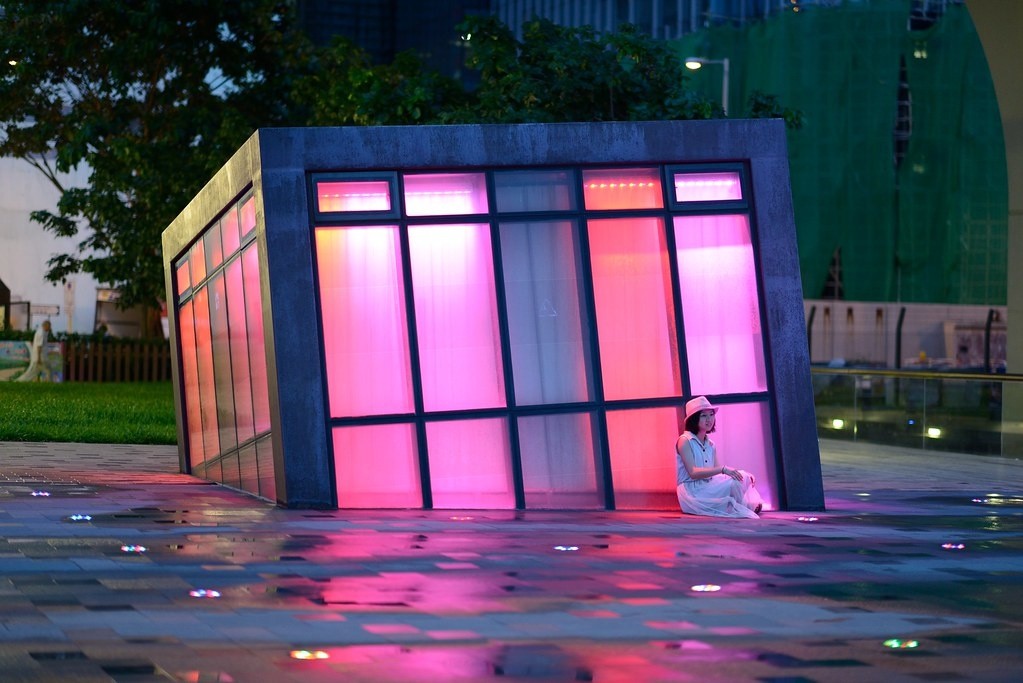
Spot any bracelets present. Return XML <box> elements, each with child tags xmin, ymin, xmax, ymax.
<box><xmin>722</xmin><ymin>466</ymin><xmax>725</xmax><ymax>474</ymax></box>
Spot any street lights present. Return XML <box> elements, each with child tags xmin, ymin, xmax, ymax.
<box><xmin>684</xmin><ymin>56</ymin><xmax>730</xmax><ymax>118</ymax></box>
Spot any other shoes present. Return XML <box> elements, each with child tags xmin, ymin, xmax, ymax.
<box><xmin>754</xmin><ymin>504</ymin><xmax>762</xmax><ymax>514</ymax></box>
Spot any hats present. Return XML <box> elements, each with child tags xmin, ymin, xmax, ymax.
<box><xmin>684</xmin><ymin>396</ymin><xmax>719</xmax><ymax>423</ymax></box>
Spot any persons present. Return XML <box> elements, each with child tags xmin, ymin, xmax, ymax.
<box><xmin>957</xmin><ymin>345</ymin><xmax>971</xmax><ymax>366</ymax></box>
<box><xmin>676</xmin><ymin>395</ymin><xmax>763</xmax><ymax>519</ymax></box>
<box><xmin>15</xmin><ymin>321</ymin><xmax>51</xmax><ymax>381</ymax></box>
